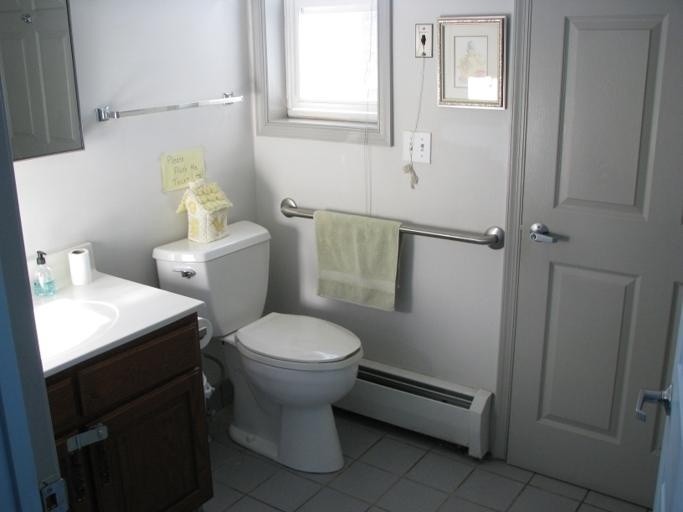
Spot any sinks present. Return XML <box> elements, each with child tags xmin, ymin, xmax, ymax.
<box><xmin>34</xmin><ymin>301</ymin><xmax>117</xmax><ymax>354</ymax></box>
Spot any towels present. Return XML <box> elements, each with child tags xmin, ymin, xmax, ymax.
<box><xmin>310</xmin><ymin>206</ymin><xmax>403</xmax><ymax>313</ymax></box>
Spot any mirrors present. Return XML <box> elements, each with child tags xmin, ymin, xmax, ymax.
<box><xmin>0</xmin><ymin>1</ymin><xmax>86</xmax><ymax>164</ymax></box>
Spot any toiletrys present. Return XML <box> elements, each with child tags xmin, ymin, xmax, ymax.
<box><xmin>32</xmin><ymin>250</ymin><xmax>58</xmax><ymax>297</ymax></box>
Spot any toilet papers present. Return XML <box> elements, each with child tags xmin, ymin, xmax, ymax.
<box><xmin>68</xmin><ymin>249</ymin><xmax>93</xmax><ymax>284</ymax></box>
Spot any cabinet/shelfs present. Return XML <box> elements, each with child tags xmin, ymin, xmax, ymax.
<box><xmin>43</xmin><ymin>323</ymin><xmax>214</xmax><ymax>510</ymax></box>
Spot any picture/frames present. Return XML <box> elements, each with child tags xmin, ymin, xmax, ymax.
<box><xmin>435</xmin><ymin>15</ymin><xmax>509</xmax><ymax>113</ymax></box>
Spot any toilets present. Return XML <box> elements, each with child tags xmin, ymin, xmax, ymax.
<box><xmin>153</xmin><ymin>221</ymin><xmax>365</xmax><ymax>474</ymax></box>
